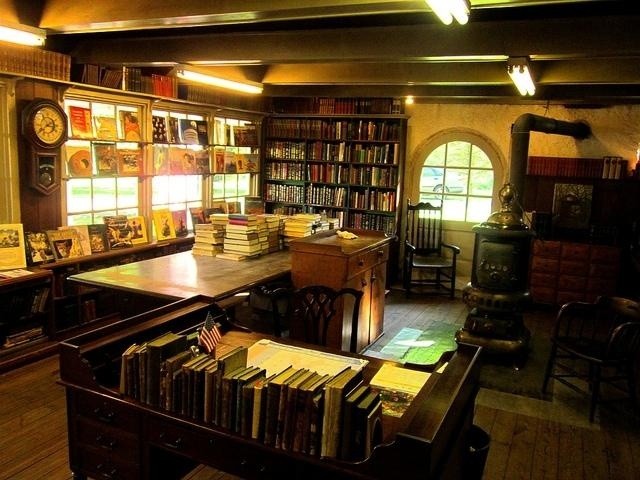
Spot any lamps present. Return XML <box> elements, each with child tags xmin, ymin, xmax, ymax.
<box><xmin>0</xmin><ymin>22</ymin><xmax>48</xmax><ymax>53</ymax></box>
<box><xmin>507</xmin><ymin>57</ymin><xmax>538</xmax><ymax>98</ymax></box>
<box><xmin>168</xmin><ymin>64</ymin><xmax>265</xmax><ymax>97</ymax></box>
<box><xmin>423</xmin><ymin>0</ymin><xmax>471</xmax><ymax>28</ymax></box>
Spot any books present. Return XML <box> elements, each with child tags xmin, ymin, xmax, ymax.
<box><xmin>263</xmin><ymin>95</ymin><xmax>400</xmax><ymax>232</ymax></box>
<box><xmin>1</xmin><ymin>40</ymin><xmax>263</xmax><ymax>111</ymax></box>
<box><xmin>1</xmin><ymin>207</ymin><xmax>188</xmax><ymax>270</ymax></box>
<box><xmin>116</xmin><ymin>330</ymin><xmax>431</xmax><ymax>464</ymax></box>
<box><xmin>190</xmin><ymin>196</ymin><xmax>340</xmax><ymax>261</ymax></box>
<box><xmin>3</xmin><ymin>280</ymin><xmax>50</xmax><ymax>347</ymax></box>
<box><xmin>527</xmin><ymin>155</ymin><xmax>627</xmax><ymax>179</ymax></box>
<box><xmin>69</xmin><ymin>103</ymin><xmax>260</xmax><ymax>175</ymax></box>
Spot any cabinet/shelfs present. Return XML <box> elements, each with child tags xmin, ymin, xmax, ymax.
<box><xmin>288</xmin><ymin>231</ymin><xmax>393</xmax><ymax>355</ymax></box>
<box><xmin>260</xmin><ymin>113</ymin><xmax>411</xmax><ymax>286</ymax></box>
<box><xmin>0</xmin><ymin>71</ymin><xmax>265</xmax><ymax>373</ymax></box>
<box><xmin>522</xmin><ymin>158</ymin><xmax>639</xmax><ymax>298</ymax></box>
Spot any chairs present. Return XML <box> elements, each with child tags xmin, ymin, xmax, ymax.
<box><xmin>405</xmin><ymin>200</ymin><xmax>462</xmax><ymax>299</ymax></box>
<box><xmin>541</xmin><ymin>297</ymin><xmax>638</xmax><ymax>424</ymax></box>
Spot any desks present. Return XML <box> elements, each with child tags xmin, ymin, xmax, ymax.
<box><xmin>68</xmin><ymin>251</ymin><xmax>290</xmax><ymax>322</ymax></box>
<box><xmin>56</xmin><ymin>295</ymin><xmax>484</xmax><ymax>480</ymax></box>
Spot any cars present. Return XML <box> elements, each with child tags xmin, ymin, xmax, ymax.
<box><xmin>419</xmin><ymin>167</ymin><xmax>465</xmax><ymax>200</ymax></box>
